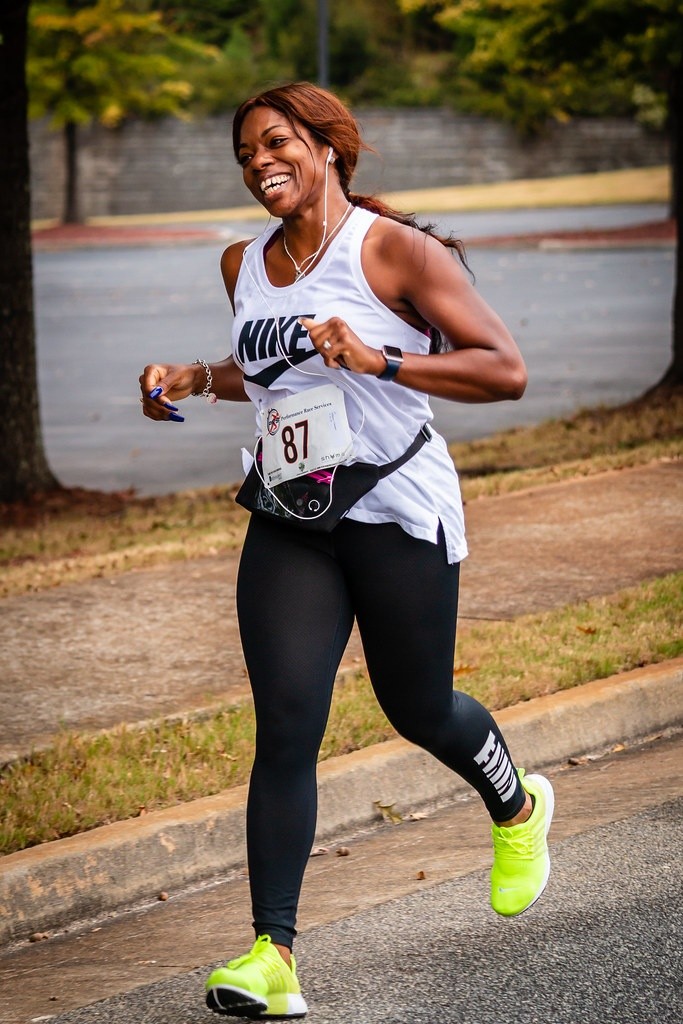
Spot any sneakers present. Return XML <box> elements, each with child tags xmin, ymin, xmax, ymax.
<box><xmin>205</xmin><ymin>934</ymin><xmax>307</xmax><ymax>1019</ymax></box>
<box><xmin>490</xmin><ymin>766</ymin><xmax>555</xmax><ymax>916</ymax></box>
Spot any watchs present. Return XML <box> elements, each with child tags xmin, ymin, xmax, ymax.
<box><xmin>376</xmin><ymin>345</ymin><xmax>404</xmax><ymax>381</ymax></box>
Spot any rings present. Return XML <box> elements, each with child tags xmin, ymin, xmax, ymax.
<box><xmin>323</xmin><ymin>340</ymin><xmax>332</xmax><ymax>351</ymax></box>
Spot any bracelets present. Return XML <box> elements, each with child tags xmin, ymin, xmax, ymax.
<box><xmin>190</xmin><ymin>358</ymin><xmax>216</xmax><ymax>404</ymax></box>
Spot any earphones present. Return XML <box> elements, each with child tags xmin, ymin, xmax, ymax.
<box><xmin>326</xmin><ymin>146</ymin><xmax>333</xmax><ymax>163</ymax></box>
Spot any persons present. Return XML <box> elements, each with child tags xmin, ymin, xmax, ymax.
<box><xmin>138</xmin><ymin>82</ymin><xmax>554</xmax><ymax>1022</ymax></box>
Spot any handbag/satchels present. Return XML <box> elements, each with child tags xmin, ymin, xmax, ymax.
<box><xmin>233</xmin><ymin>425</ymin><xmax>434</xmax><ymax>532</ymax></box>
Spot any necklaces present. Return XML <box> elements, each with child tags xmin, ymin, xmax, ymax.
<box><xmin>283</xmin><ymin>202</ymin><xmax>352</xmax><ymax>283</ymax></box>
